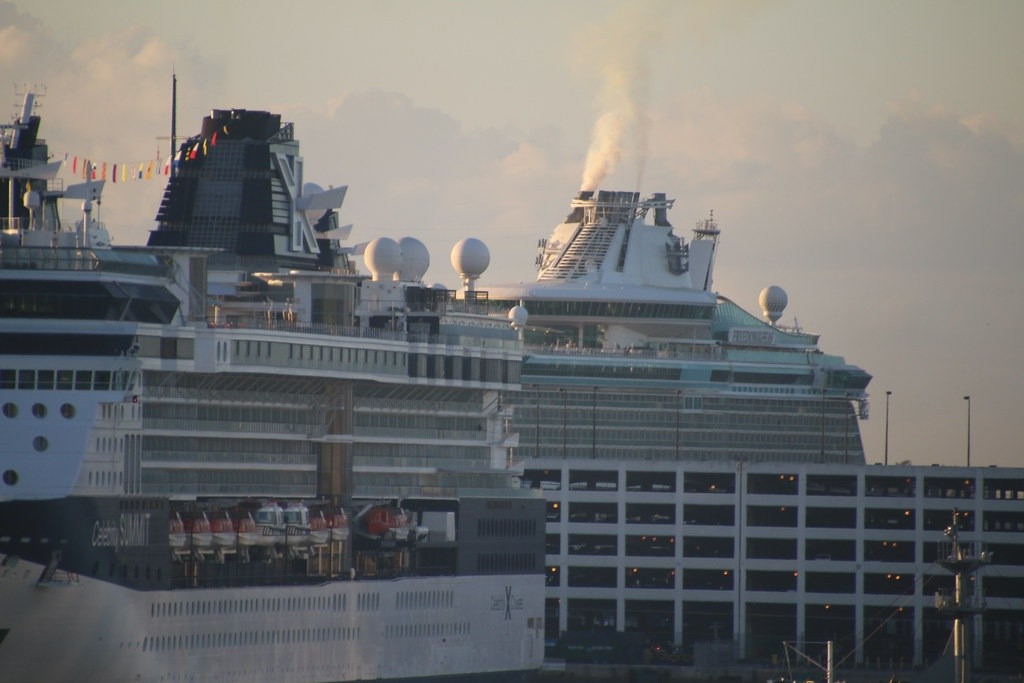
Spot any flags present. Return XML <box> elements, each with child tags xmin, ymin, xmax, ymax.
<box><xmin>64</xmin><ymin>126</ymin><xmax>229</xmax><ymax>183</ymax></box>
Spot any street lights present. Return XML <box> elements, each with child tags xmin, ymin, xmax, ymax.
<box><xmin>845</xmin><ymin>393</ymin><xmax>849</xmax><ymax>464</ymax></box>
<box><xmin>963</xmin><ymin>396</ymin><xmax>970</xmax><ymax>468</ymax></box>
<box><xmin>821</xmin><ymin>390</ymin><xmax>826</xmax><ymax>464</ymax></box>
<box><xmin>592</xmin><ymin>387</ymin><xmax>598</xmax><ymax>459</ymax></box>
<box><xmin>674</xmin><ymin>389</ymin><xmax>682</xmax><ymax>460</ymax></box>
<box><xmin>531</xmin><ymin>384</ymin><xmax>539</xmax><ymax>456</ymax></box>
<box><xmin>559</xmin><ymin>388</ymin><xmax>567</xmax><ymax>461</ymax></box>
<box><xmin>884</xmin><ymin>390</ymin><xmax>893</xmax><ymax>463</ymax></box>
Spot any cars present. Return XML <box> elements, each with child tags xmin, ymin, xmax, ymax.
<box><xmin>555</xmin><ymin>626</ymin><xmax>672</xmax><ymax>663</ymax></box>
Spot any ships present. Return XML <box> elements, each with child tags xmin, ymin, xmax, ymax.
<box><xmin>1</xmin><ymin>91</ymin><xmax>545</xmax><ymax>683</ymax></box>
<box><xmin>451</xmin><ymin>192</ymin><xmax>873</xmax><ymax>464</ymax></box>
